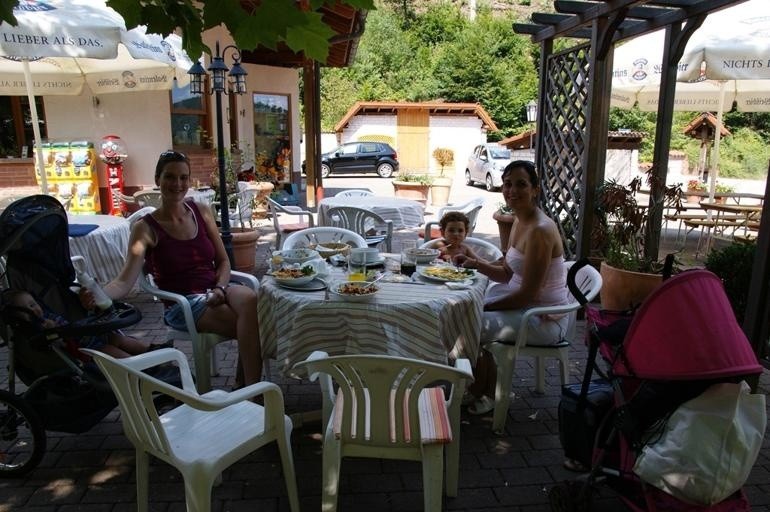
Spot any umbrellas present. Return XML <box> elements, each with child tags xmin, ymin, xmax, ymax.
<box><xmin>0</xmin><ymin>0</ymin><xmax>207</xmax><ymax>196</ymax></box>
<box><xmin>572</xmin><ymin>1</ymin><xmax>770</xmax><ymax>220</ymax></box>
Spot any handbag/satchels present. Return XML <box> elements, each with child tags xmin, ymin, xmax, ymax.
<box><xmin>559</xmin><ymin>378</ymin><xmax>614</xmax><ymax>461</ymax></box>
<box><xmin>633</xmin><ymin>380</ymin><xmax>767</xmax><ymax>506</ymax></box>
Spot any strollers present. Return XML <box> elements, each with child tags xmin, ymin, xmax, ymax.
<box><xmin>0</xmin><ymin>195</ymin><xmax>195</xmax><ymax>477</ymax></box>
<box><xmin>558</xmin><ymin>253</ymin><xmax>767</xmax><ymax>512</ymax></box>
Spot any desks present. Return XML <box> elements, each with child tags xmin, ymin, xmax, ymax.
<box><xmin>636</xmin><ymin>189</ymin><xmax>764</xmax><ymax>256</ymax></box>
<box><xmin>64</xmin><ymin>207</ymin><xmax>140</xmax><ymax>300</ymax></box>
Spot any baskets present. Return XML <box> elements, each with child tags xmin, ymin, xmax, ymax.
<box><xmin>310</xmin><ymin>243</ymin><xmax>351</xmax><ymax>260</ymax></box>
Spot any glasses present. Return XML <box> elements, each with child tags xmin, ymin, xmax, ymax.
<box><xmin>162</xmin><ymin>149</ymin><xmax>186</xmax><ymax>160</ymax></box>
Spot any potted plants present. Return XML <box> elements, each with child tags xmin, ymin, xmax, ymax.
<box><xmin>593</xmin><ymin>167</ymin><xmax>687</xmax><ymax>312</ymax></box>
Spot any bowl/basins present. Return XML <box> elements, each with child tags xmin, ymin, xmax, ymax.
<box><xmin>351</xmin><ymin>248</ymin><xmax>379</xmax><ymax>262</ymax></box>
<box><xmin>271</xmin><ymin>248</ymin><xmax>319</xmax><ymax>264</ymax></box>
<box><xmin>272</xmin><ymin>268</ymin><xmax>318</xmax><ymax>286</ymax></box>
<box><xmin>402</xmin><ymin>248</ymin><xmax>441</xmax><ymax>264</ymax></box>
<box><xmin>329</xmin><ymin>281</ymin><xmax>385</xmax><ymax>302</ymax></box>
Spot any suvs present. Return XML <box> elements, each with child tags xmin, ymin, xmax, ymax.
<box><xmin>302</xmin><ymin>142</ymin><xmax>399</xmax><ymax>178</ymax></box>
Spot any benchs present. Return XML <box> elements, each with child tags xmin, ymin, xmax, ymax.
<box><xmin>630</xmin><ymin>204</ymin><xmax>763</xmax><ymax>262</ymax></box>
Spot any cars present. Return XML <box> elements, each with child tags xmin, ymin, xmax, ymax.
<box><xmin>465</xmin><ymin>143</ymin><xmax>512</xmax><ymax>192</ymax></box>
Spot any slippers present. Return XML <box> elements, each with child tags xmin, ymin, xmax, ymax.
<box><xmin>462</xmin><ymin>389</ymin><xmax>515</xmax><ymax>415</ymax></box>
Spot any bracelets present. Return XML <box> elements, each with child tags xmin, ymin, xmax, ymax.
<box><xmin>472</xmin><ymin>258</ymin><xmax>483</xmax><ymax>268</ymax></box>
<box><xmin>213</xmin><ymin>285</ymin><xmax>228</xmax><ymax>295</ymax></box>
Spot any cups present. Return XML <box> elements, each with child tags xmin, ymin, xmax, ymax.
<box><xmin>347</xmin><ymin>250</ymin><xmax>366</xmax><ymax>283</ymax></box>
<box><xmin>399</xmin><ymin>239</ymin><xmax>417</xmax><ymax>273</ymax></box>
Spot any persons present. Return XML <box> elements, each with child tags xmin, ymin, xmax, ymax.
<box><xmin>431</xmin><ymin>211</ymin><xmax>506</xmax><ymax>270</ymax></box>
<box><xmin>81</xmin><ymin>148</ymin><xmax>263</xmax><ymax>405</ymax></box>
<box><xmin>2</xmin><ymin>288</ymin><xmax>176</xmax><ymax>377</ymax></box>
<box><xmin>453</xmin><ymin>159</ymin><xmax>572</xmax><ymax>414</ymax></box>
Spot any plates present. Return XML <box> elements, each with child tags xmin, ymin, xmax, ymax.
<box><xmin>349</xmin><ymin>256</ymin><xmax>387</xmax><ymax>266</ymax></box>
<box><xmin>418</xmin><ymin>267</ymin><xmax>478</xmax><ymax>281</ymax></box>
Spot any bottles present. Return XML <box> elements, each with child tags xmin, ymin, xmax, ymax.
<box><xmin>75</xmin><ymin>266</ymin><xmax>113</xmax><ymax>310</ymax></box>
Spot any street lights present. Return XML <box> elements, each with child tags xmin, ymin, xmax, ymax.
<box><xmin>525</xmin><ymin>100</ymin><xmax>538</xmax><ymax>162</ymax></box>
<box><xmin>189</xmin><ymin>40</ymin><xmax>247</xmax><ymax>271</ymax></box>
